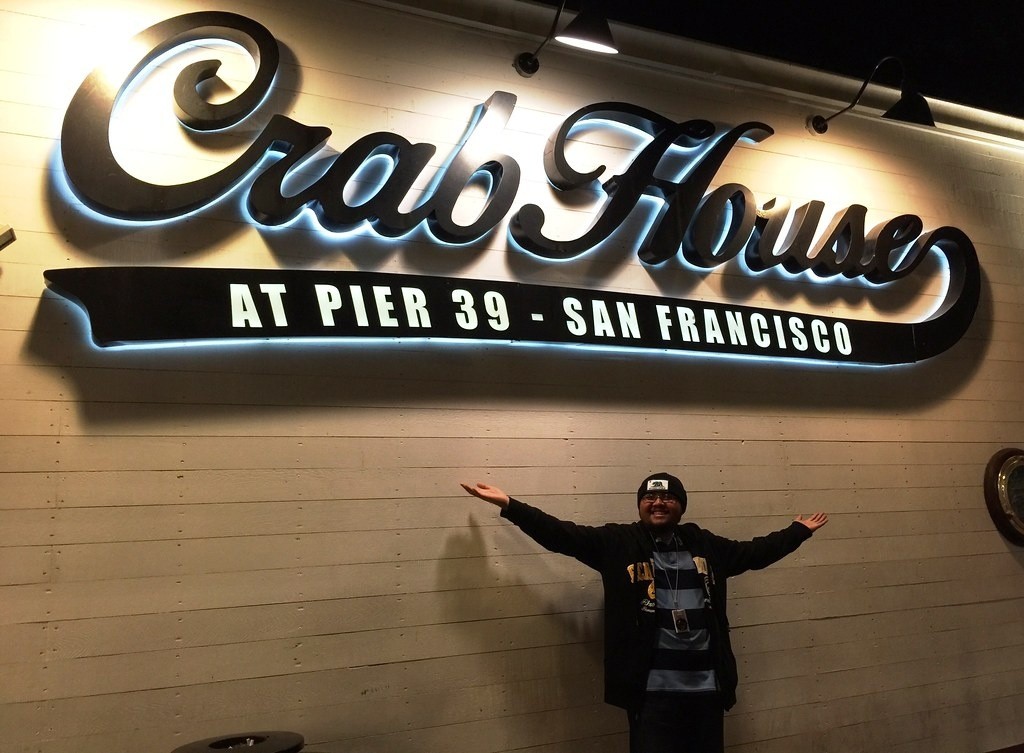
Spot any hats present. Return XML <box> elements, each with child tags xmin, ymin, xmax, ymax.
<box><xmin>637</xmin><ymin>472</ymin><xmax>687</xmax><ymax>515</ymax></box>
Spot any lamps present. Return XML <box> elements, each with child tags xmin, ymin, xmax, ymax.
<box><xmin>812</xmin><ymin>53</ymin><xmax>939</xmax><ymax>134</ymax></box>
<box><xmin>512</xmin><ymin>0</ymin><xmax>619</xmax><ymax>79</ymax></box>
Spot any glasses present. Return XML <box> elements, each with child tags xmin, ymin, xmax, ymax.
<box><xmin>642</xmin><ymin>492</ymin><xmax>678</xmax><ymax>503</ymax></box>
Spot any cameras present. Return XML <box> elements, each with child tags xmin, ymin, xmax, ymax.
<box><xmin>672</xmin><ymin>608</ymin><xmax>690</xmax><ymax>633</ymax></box>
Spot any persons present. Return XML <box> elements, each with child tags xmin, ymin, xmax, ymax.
<box><xmin>461</xmin><ymin>473</ymin><xmax>829</xmax><ymax>753</ymax></box>
